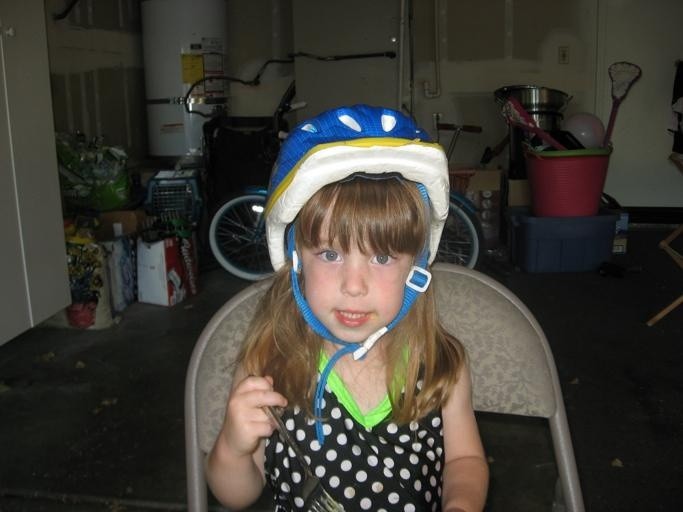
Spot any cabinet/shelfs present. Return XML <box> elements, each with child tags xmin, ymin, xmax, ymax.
<box><xmin>0</xmin><ymin>2</ymin><xmax>69</xmax><ymax>350</ymax></box>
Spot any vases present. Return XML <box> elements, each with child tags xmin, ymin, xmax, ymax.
<box><xmin>65</xmin><ymin>299</ymin><xmax>97</xmax><ymax>329</ymax></box>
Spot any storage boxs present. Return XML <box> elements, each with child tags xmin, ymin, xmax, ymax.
<box><xmin>510</xmin><ymin>200</ymin><xmax>620</xmax><ymax>275</ymax></box>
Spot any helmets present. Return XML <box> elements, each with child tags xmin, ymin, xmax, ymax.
<box><xmin>263</xmin><ymin>104</ymin><xmax>451</xmax><ymax>272</ymax></box>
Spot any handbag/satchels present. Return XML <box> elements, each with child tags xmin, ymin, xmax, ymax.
<box><xmin>47</xmin><ymin>230</ymin><xmax>200</xmax><ymax>331</ymax></box>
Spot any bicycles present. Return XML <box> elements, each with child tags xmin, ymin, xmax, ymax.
<box><xmin>207</xmin><ymin>100</ymin><xmax>485</xmax><ymax>283</ymax></box>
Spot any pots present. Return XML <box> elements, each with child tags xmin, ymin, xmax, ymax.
<box><xmin>528</xmin><ymin>111</ymin><xmax>564</xmax><ymax>145</ymax></box>
<box><xmin>493</xmin><ymin>84</ymin><xmax>574</xmax><ymax>114</ymax></box>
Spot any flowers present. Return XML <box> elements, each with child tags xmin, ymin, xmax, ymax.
<box><xmin>59</xmin><ymin>220</ymin><xmax>103</xmax><ymax>309</ymax></box>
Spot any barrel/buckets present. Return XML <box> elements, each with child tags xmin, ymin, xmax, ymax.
<box><xmin>527</xmin><ymin>145</ymin><xmax>612</xmax><ymax>217</ymax></box>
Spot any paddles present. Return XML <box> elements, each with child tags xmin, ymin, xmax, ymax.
<box><xmin>602</xmin><ymin>62</ymin><xmax>642</xmax><ymax>148</ymax></box>
<box><xmin>500</xmin><ymin>98</ymin><xmax>568</xmax><ymax>151</ymax></box>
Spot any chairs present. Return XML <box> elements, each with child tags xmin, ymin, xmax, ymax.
<box><xmin>180</xmin><ymin>260</ymin><xmax>586</xmax><ymax>512</ymax></box>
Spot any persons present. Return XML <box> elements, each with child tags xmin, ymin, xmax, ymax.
<box><xmin>202</xmin><ymin>106</ymin><xmax>489</xmax><ymax>511</ymax></box>
